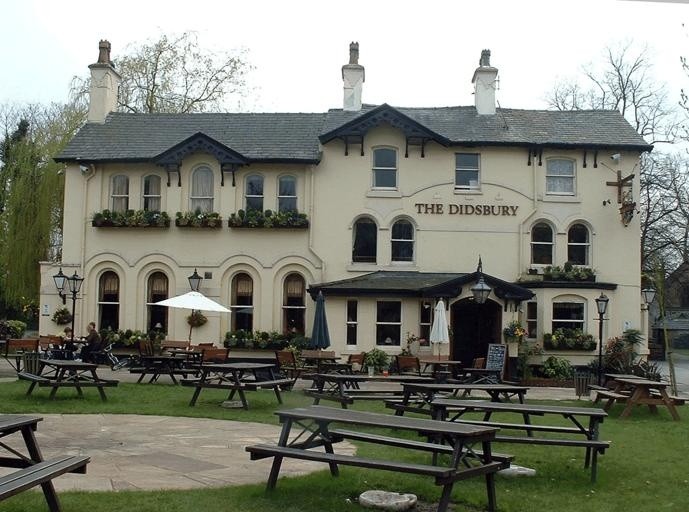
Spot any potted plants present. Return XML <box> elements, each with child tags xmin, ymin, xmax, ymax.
<box><xmin>541</xmin><ymin>262</ymin><xmax>596</xmax><ymax>283</ymax></box>
<box><xmin>90</xmin><ymin>208</ymin><xmax>310</xmax><ymax>229</ymax></box>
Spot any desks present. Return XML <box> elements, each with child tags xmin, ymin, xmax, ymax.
<box><xmin>2</xmin><ymin>413</ymin><xmax>62</xmax><ymax>510</ymax></box>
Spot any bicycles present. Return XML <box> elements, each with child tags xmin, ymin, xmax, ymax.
<box><xmin>103</xmin><ymin>342</ymin><xmax>140</xmax><ymax>371</ymax></box>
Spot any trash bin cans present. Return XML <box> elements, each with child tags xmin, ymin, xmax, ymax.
<box><xmin>574</xmin><ymin>365</ymin><xmax>593</xmax><ymax>396</ymax></box>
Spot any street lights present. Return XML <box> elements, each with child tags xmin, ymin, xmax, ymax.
<box><xmin>593</xmin><ymin>291</ymin><xmax>610</xmax><ymax>386</ymax></box>
<box><xmin>68</xmin><ymin>270</ymin><xmax>84</xmax><ymax>344</ymax></box>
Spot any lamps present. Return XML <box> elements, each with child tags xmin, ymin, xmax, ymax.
<box><xmin>187</xmin><ymin>267</ymin><xmax>203</xmax><ymax>292</ymax></box>
<box><xmin>53</xmin><ymin>267</ymin><xmax>68</xmax><ymax>305</ymax></box>
<box><xmin>469</xmin><ymin>253</ymin><xmax>494</xmax><ymax>306</ymax></box>
<box><xmin>640</xmin><ymin>285</ymin><xmax>656</xmax><ymax>311</ymax></box>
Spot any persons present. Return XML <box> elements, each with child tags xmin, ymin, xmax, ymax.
<box><xmin>56</xmin><ymin>321</ymin><xmax>115</xmax><ymax>363</ymax></box>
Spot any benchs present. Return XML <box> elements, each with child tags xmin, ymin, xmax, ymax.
<box><xmin>244</xmin><ymin>399</ymin><xmax>610</xmax><ymax>511</ymax></box>
<box><xmin>1</xmin><ymin>455</ymin><xmax>91</xmax><ymax>510</ymax></box>
<box><xmin>1</xmin><ymin>337</ymin><xmax>530</xmax><ymax>411</ymax></box>
<box><xmin>588</xmin><ymin>373</ymin><xmax>687</xmax><ymax>424</ymax></box>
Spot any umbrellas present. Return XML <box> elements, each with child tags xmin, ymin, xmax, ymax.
<box><xmin>430</xmin><ymin>297</ymin><xmax>451</xmax><ymax>359</ymax></box>
<box><xmin>145</xmin><ymin>289</ymin><xmax>232</xmax><ymax>361</ymax></box>
<box><xmin>310</xmin><ymin>290</ymin><xmax>330</xmax><ymax>350</ymax></box>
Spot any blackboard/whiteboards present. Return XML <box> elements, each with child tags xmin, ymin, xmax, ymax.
<box><xmin>484</xmin><ymin>344</ymin><xmax>508</xmax><ymax>380</ymax></box>
